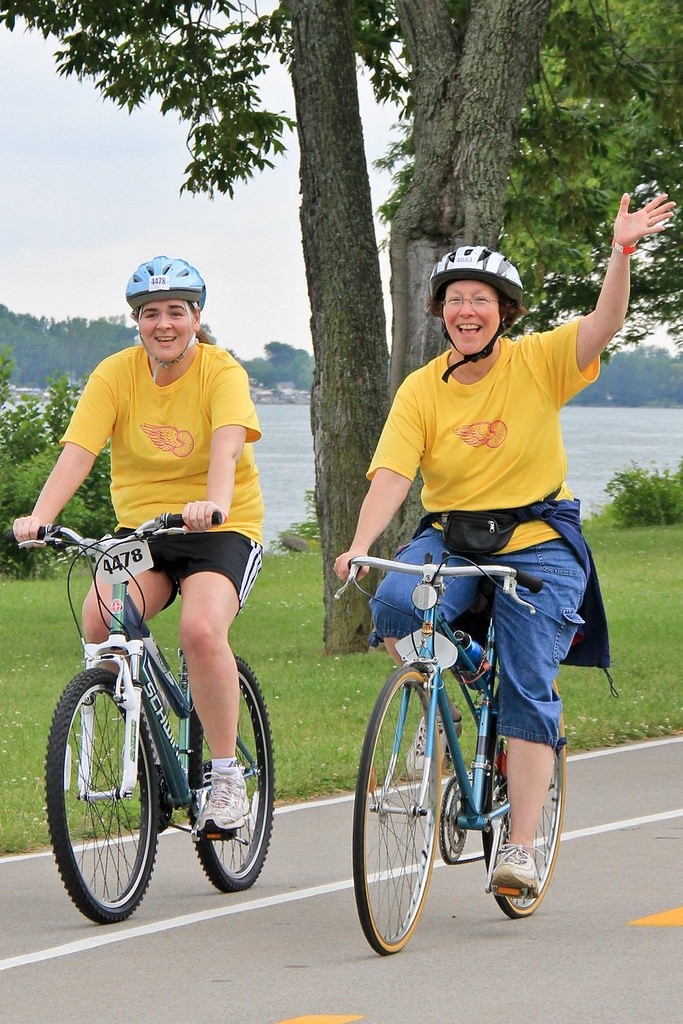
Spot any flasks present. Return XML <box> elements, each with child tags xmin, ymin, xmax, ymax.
<box><xmin>452</xmin><ymin>632</ymin><xmax>493</xmax><ymax>690</ymax></box>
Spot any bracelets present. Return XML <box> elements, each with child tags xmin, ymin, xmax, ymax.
<box><xmin>612</xmin><ymin>238</ymin><xmax>636</xmax><ymax>254</ymax></box>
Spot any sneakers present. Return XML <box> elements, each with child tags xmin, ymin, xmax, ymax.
<box><xmin>195</xmin><ymin>765</ymin><xmax>250</xmax><ymax>833</ymax></box>
<box><xmin>490</xmin><ymin>850</ymin><xmax>540</xmax><ymax>900</ymax></box>
<box><xmin>406</xmin><ymin>714</ymin><xmax>461</xmax><ymax>779</ymax></box>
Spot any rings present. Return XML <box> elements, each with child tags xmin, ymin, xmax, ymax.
<box><xmin>648</xmin><ymin>219</ymin><xmax>654</xmax><ymax>225</ymax></box>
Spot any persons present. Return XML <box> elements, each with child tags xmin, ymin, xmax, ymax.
<box><xmin>12</xmin><ymin>256</ymin><xmax>266</xmax><ymax>835</ymax></box>
<box><xmin>333</xmin><ymin>191</ymin><xmax>676</xmax><ymax>890</ymax></box>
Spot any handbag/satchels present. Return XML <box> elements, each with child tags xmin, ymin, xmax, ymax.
<box><xmin>440</xmin><ymin>510</ymin><xmax>521</xmax><ymax>555</ymax></box>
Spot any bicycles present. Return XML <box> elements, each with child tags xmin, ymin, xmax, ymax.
<box><xmin>335</xmin><ymin>550</ymin><xmax>567</xmax><ymax>956</ymax></box>
<box><xmin>1</xmin><ymin>510</ymin><xmax>276</xmax><ymax>924</ymax></box>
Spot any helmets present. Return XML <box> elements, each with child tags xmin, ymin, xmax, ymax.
<box><xmin>429</xmin><ymin>245</ymin><xmax>524</xmax><ymax>306</ymax></box>
<box><xmin>125</xmin><ymin>256</ymin><xmax>207</xmax><ymax>311</ymax></box>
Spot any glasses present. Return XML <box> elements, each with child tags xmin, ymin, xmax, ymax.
<box><xmin>440</xmin><ymin>296</ymin><xmax>499</xmax><ymax>306</ymax></box>
